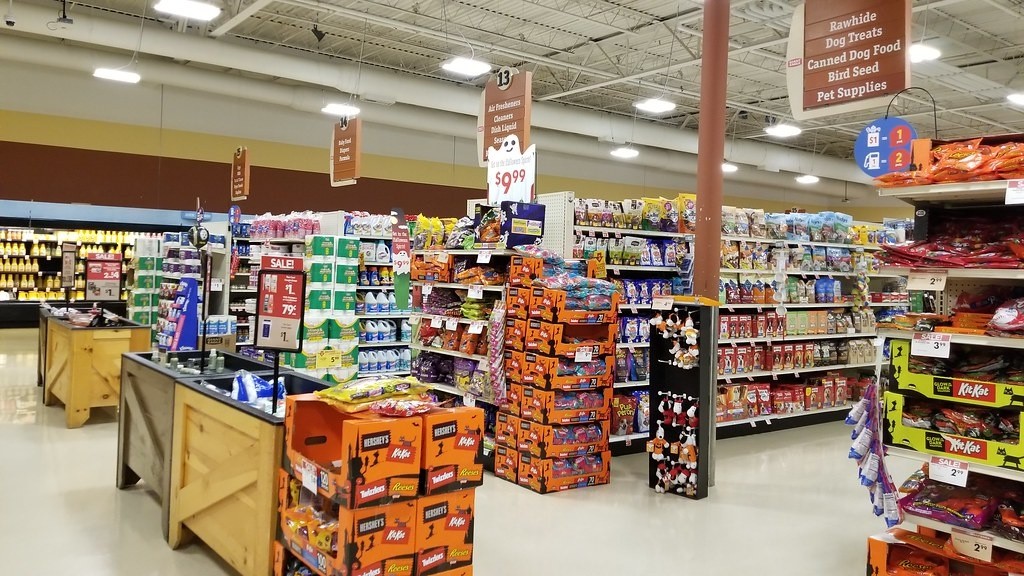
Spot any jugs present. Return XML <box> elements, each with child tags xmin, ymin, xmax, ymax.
<box><xmin>18</xmin><ymin>290</ymin><xmax>65</xmax><ymax>300</ymax></box>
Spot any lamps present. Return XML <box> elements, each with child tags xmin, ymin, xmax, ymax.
<box><xmin>154</xmin><ymin>0</ymin><xmax>221</xmax><ymax>22</ymax></box>
<box><xmin>609</xmin><ymin>147</ymin><xmax>640</xmax><ymax>159</ymax></box>
<box><xmin>438</xmin><ymin>56</ymin><xmax>492</xmax><ymax>78</ymax></box>
<box><xmin>91</xmin><ymin>66</ymin><xmax>143</xmax><ymax>83</ymax></box>
<box><xmin>763</xmin><ymin>123</ymin><xmax>802</xmax><ymax>138</ymax></box>
<box><xmin>722</xmin><ymin>161</ymin><xmax>738</xmax><ymax>172</ymax></box>
<box><xmin>793</xmin><ymin>174</ymin><xmax>819</xmax><ymax>184</ymax></box>
<box><xmin>631</xmin><ymin>98</ymin><xmax>676</xmax><ymax>113</ymax></box>
<box><xmin>909</xmin><ymin>42</ymin><xmax>941</xmax><ymax>64</ymax></box>
<box><xmin>320</xmin><ymin>102</ymin><xmax>361</xmax><ymax>118</ymax></box>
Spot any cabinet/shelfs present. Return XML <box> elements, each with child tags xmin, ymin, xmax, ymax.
<box><xmin>1</xmin><ymin>190</ymin><xmax>914</xmax><ymax>472</ymax></box>
<box><xmin>877</xmin><ymin>130</ymin><xmax>1024</xmax><ymax>554</ymax></box>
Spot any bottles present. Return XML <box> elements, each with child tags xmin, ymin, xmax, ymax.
<box><xmin>0</xmin><ymin>228</ymin><xmax>161</xmax><ymax>287</ymax></box>
<box><xmin>151</xmin><ymin>341</ymin><xmax>225</xmax><ymax>376</ymax></box>
<box><xmin>357</xmin><ymin>240</ymin><xmax>412</xmax><ymax>373</ymax></box>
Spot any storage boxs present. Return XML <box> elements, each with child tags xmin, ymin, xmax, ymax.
<box><xmin>271</xmin><ymin>392</ymin><xmax>483</xmax><ymax>576</ymax></box>
<box><xmin>494</xmin><ymin>255</ymin><xmax>847</xmax><ymax>494</ymax></box>
<box><xmin>866</xmin><ymin>340</ymin><xmax>1024</xmax><ymax>576</ymax></box>
